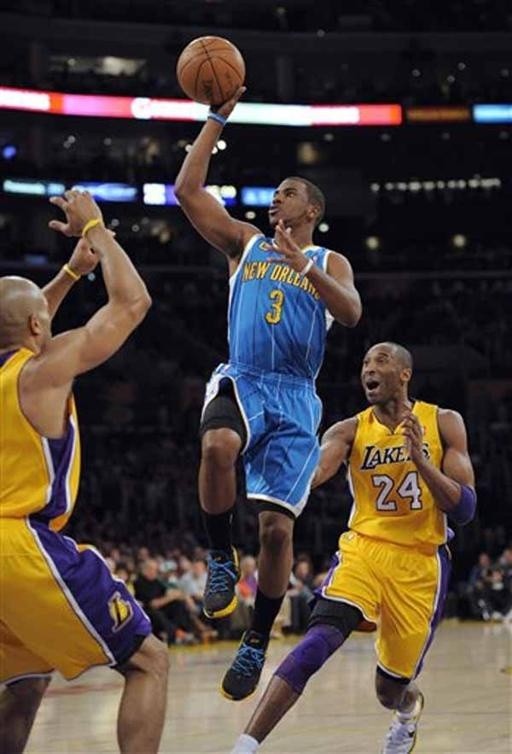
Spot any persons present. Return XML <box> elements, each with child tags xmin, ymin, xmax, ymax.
<box><xmin>175</xmin><ymin>87</ymin><xmax>365</xmax><ymax>700</ymax></box>
<box><xmin>89</xmin><ymin>535</ymin><xmax>338</xmax><ymax>646</ymax></box>
<box><xmin>444</xmin><ymin>549</ymin><xmax>512</xmax><ymax>622</ymax></box>
<box><xmin>235</xmin><ymin>342</ymin><xmax>482</xmax><ymax>753</ymax></box>
<box><xmin>0</xmin><ymin>188</ymin><xmax>173</xmax><ymax>754</ymax></box>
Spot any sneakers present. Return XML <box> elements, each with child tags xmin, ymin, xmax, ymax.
<box><xmin>166</xmin><ymin>628</ymin><xmax>217</xmax><ymax>647</ymax></box>
<box><xmin>202</xmin><ymin>547</ymin><xmax>240</xmax><ymax>618</ymax></box>
<box><xmin>381</xmin><ymin>688</ymin><xmax>424</xmax><ymax>753</ymax></box>
<box><xmin>219</xmin><ymin>630</ymin><xmax>267</xmax><ymax>702</ymax></box>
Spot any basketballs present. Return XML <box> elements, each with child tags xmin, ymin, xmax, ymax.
<box><xmin>177</xmin><ymin>35</ymin><xmax>245</xmax><ymax>105</ymax></box>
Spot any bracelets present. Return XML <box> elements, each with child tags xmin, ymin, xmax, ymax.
<box><xmin>64</xmin><ymin>264</ymin><xmax>81</xmax><ymax>281</ymax></box>
<box><xmin>80</xmin><ymin>218</ymin><xmax>99</xmax><ymax>240</ymax></box>
<box><xmin>297</xmin><ymin>258</ymin><xmax>313</xmax><ymax>278</ymax></box>
<box><xmin>206</xmin><ymin>114</ymin><xmax>226</xmax><ymax>126</ymax></box>
